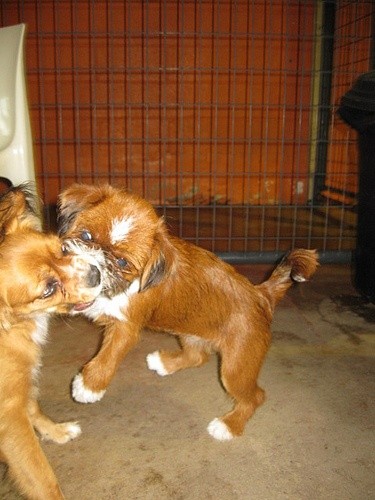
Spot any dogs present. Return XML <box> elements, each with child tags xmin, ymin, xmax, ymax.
<box><xmin>0</xmin><ymin>175</ymin><xmax>320</xmax><ymax>499</ymax></box>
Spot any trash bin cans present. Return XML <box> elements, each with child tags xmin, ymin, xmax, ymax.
<box><xmin>336</xmin><ymin>70</ymin><xmax>375</xmax><ymax>302</ymax></box>
